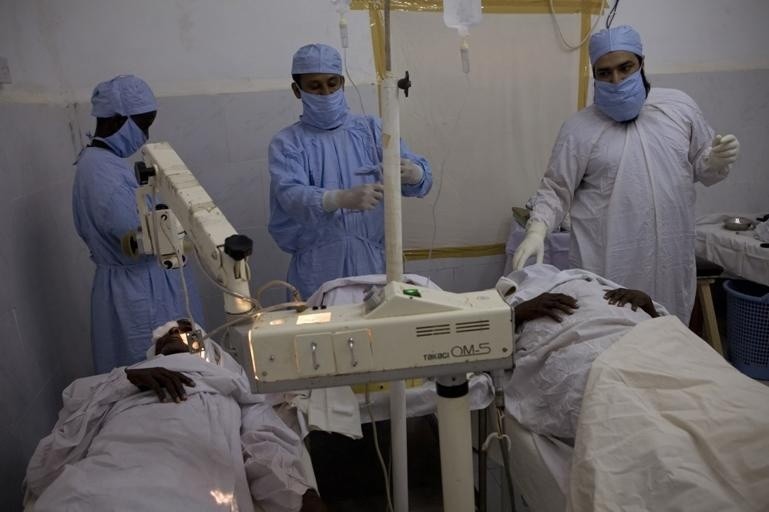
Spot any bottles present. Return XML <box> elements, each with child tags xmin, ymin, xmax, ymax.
<box><xmin>443</xmin><ymin>1</ymin><xmax>482</xmax><ymax>39</ymax></box>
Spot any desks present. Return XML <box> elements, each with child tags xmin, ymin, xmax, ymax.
<box><xmin>693</xmin><ymin>212</ymin><xmax>768</xmax><ymax>326</ymax></box>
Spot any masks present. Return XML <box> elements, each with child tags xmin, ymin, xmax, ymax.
<box><xmin>294</xmin><ymin>80</ymin><xmax>350</xmax><ymax>130</ymax></box>
<box><xmin>93</xmin><ymin>117</ymin><xmax>147</xmax><ymax>157</ymax></box>
<box><xmin>594</xmin><ymin>66</ymin><xmax>646</xmax><ymax>122</ymax></box>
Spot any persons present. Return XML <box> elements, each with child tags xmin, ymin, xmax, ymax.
<box><xmin>268</xmin><ymin>44</ymin><xmax>433</xmax><ymax>312</ymax></box>
<box><xmin>512</xmin><ymin>25</ymin><xmax>741</xmax><ymax>327</ymax></box>
<box><xmin>21</xmin><ymin>318</ymin><xmax>326</xmax><ymax>512</ymax></box>
<box><xmin>497</xmin><ymin>263</ymin><xmax>769</xmax><ymax>512</ymax></box>
<box><xmin>71</xmin><ymin>76</ymin><xmax>205</xmax><ymax>375</ymax></box>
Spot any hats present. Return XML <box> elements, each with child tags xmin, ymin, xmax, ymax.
<box><xmin>291</xmin><ymin>43</ymin><xmax>342</xmax><ymax>76</ymax></box>
<box><xmin>589</xmin><ymin>25</ymin><xmax>643</xmax><ymax>66</ymax></box>
<box><xmin>91</xmin><ymin>74</ymin><xmax>157</xmax><ymax>119</ymax></box>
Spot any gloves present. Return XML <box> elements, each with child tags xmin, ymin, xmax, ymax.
<box><xmin>712</xmin><ymin>134</ymin><xmax>740</xmax><ymax>164</ymax></box>
<box><xmin>511</xmin><ymin>222</ymin><xmax>546</xmax><ymax>270</ymax></box>
<box><xmin>401</xmin><ymin>159</ymin><xmax>423</xmax><ymax>184</ymax></box>
<box><xmin>322</xmin><ymin>183</ymin><xmax>384</xmax><ymax>213</ymax></box>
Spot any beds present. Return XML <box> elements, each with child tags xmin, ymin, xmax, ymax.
<box><xmin>504</xmin><ymin>380</ymin><xmax>606</xmax><ymax>511</ymax></box>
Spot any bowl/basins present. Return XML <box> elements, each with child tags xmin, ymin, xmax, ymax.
<box><xmin>724</xmin><ymin>216</ymin><xmax>754</xmax><ymax>231</ymax></box>
